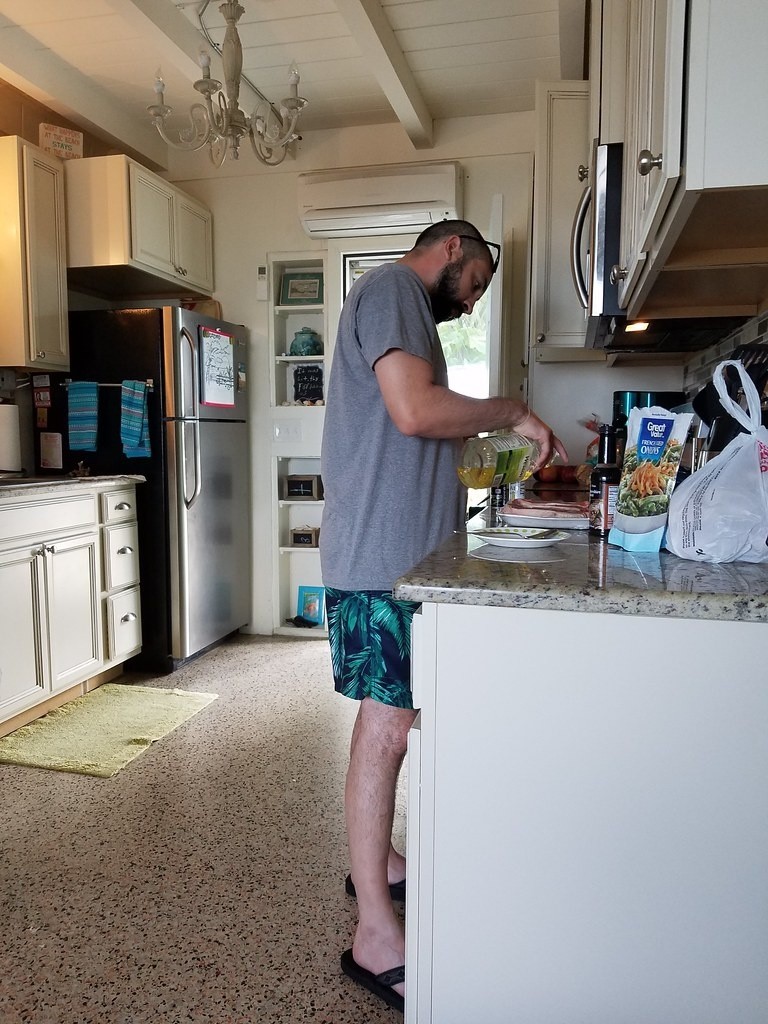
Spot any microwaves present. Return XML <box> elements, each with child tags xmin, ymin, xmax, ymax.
<box><xmin>570</xmin><ymin>146</ymin><xmax>754</xmax><ymax>351</ymax></box>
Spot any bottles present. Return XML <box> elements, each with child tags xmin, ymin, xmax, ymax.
<box><xmin>589</xmin><ymin>425</ymin><xmax>621</xmax><ymax>538</ymax></box>
<box><xmin>456</xmin><ymin>432</ymin><xmax>560</xmax><ymax>488</ymax></box>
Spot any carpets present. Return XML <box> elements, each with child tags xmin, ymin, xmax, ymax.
<box><xmin>0</xmin><ymin>682</ymin><xmax>220</xmax><ymax>780</ymax></box>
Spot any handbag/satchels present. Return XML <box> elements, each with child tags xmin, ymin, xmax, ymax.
<box><xmin>668</xmin><ymin>361</ymin><xmax>768</xmax><ymax>564</ymax></box>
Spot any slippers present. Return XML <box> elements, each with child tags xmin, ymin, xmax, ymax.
<box><xmin>340</xmin><ymin>948</ymin><xmax>405</xmax><ymax>1013</ymax></box>
<box><xmin>345</xmin><ymin>873</ymin><xmax>406</xmax><ymax>901</ymax></box>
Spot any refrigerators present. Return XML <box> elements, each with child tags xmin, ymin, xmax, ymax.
<box><xmin>31</xmin><ymin>305</ymin><xmax>251</xmax><ymax>673</ymax></box>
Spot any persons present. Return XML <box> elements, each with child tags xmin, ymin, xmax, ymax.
<box><xmin>319</xmin><ymin>220</ymin><xmax>568</xmax><ymax>1012</ymax></box>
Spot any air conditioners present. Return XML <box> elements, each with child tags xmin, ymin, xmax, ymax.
<box><xmin>296</xmin><ymin>159</ymin><xmax>466</xmax><ymax>239</ymax></box>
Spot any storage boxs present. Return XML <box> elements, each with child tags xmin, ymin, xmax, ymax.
<box><xmin>284</xmin><ymin>475</ymin><xmax>321</xmax><ymax>501</ymax></box>
<box><xmin>288</xmin><ymin>528</ymin><xmax>319</xmax><ymax>548</ymax></box>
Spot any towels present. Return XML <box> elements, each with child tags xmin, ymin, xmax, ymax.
<box><xmin>67</xmin><ymin>381</ymin><xmax>98</xmax><ymax>452</ymax></box>
<box><xmin>120</xmin><ymin>379</ymin><xmax>152</xmax><ymax>458</ymax></box>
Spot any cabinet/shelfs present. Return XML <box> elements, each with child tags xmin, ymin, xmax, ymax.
<box><xmin>394</xmin><ymin>483</ymin><xmax>768</xmax><ymax>1024</ymax></box>
<box><xmin>0</xmin><ymin>134</ymin><xmax>72</xmax><ymax>376</ymax></box>
<box><xmin>0</xmin><ymin>472</ymin><xmax>147</xmax><ymax>741</ymax></box>
<box><xmin>63</xmin><ymin>154</ymin><xmax>216</xmax><ymax>302</ymax></box>
<box><xmin>531</xmin><ymin>0</ymin><xmax>768</xmax><ymax>370</ymax></box>
<box><xmin>271</xmin><ymin>456</ymin><xmax>328</xmax><ymax>633</ymax></box>
<box><xmin>267</xmin><ymin>250</ymin><xmax>325</xmax><ymax>407</ymax></box>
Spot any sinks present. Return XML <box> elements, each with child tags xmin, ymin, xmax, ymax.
<box><xmin>0</xmin><ymin>476</ymin><xmax>81</xmax><ymax>491</ymax></box>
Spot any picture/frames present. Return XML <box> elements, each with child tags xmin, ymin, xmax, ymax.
<box><xmin>279</xmin><ymin>272</ymin><xmax>324</xmax><ymax>305</ymax></box>
<box><xmin>297</xmin><ymin>585</ymin><xmax>325</xmax><ymax>625</ymax></box>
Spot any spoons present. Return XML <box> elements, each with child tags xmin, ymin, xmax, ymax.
<box><xmin>453</xmin><ymin>529</ymin><xmax>559</xmax><ymax>539</ymax></box>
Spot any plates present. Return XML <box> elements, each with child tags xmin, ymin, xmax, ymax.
<box><xmin>472</xmin><ymin>527</ymin><xmax>572</xmax><ymax>547</ymax></box>
<box><xmin>469</xmin><ymin>543</ymin><xmax>570</xmax><ymax>563</ymax></box>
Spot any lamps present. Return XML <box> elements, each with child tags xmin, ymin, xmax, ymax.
<box><xmin>147</xmin><ymin>0</ymin><xmax>309</xmax><ymax>169</ymax></box>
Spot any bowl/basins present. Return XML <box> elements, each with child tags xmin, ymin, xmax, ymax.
<box><xmin>533</xmin><ymin>465</ymin><xmax>563</xmax><ymax>482</ymax></box>
<box><xmin>559</xmin><ymin>466</ymin><xmax>578</xmax><ymax>483</ymax></box>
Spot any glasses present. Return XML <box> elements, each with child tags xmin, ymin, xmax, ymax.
<box><xmin>460</xmin><ymin>234</ymin><xmax>501</xmax><ymax>273</ymax></box>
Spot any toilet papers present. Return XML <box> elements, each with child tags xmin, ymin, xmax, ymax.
<box><xmin>0</xmin><ymin>404</ymin><xmax>22</xmax><ymax>471</ymax></box>
